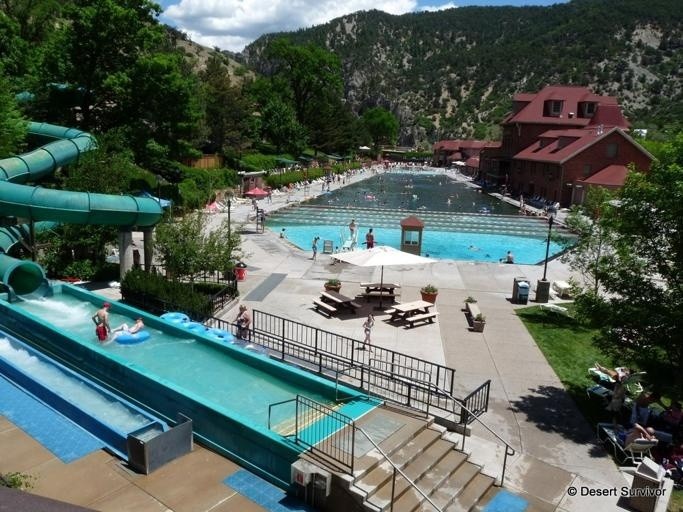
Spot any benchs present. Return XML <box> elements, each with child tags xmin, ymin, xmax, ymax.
<box><xmin>312</xmin><ymin>281</ymin><xmax>439</xmax><ymax>328</ymax></box>
<box><xmin>466</xmin><ymin>302</ymin><xmax>480</xmax><ymax>321</ymax></box>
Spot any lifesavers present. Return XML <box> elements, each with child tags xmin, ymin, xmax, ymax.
<box><xmin>115</xmin><ymin>329</ymin><xmax>149</xmax><ymax>343</ymax></box>
<box><xmin>159</xmin><ymin>312</ymin><xmax>269</xmax><ymax>357</ymax></box>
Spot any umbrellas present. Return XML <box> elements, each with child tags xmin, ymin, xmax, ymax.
<box><xmin>330</xmin><ymin>244</ymin><xmax>439</xmax><ymax>312</ymax></box>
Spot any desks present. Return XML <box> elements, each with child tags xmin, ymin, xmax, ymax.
<box><xmin>552</xmin><ymin>280</ymin><xmax>574</xmax><ymax>299</ymax></box>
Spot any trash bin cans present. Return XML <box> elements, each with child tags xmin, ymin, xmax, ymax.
<box><xmin>224</xmin><ymin>261</ymin><xmax>248</xmax><ymax>282</ymax></box>
<box><xmin>629</xmin><ymin>455</ymin><xmax>666</xmax><ymax>512</ymax></box>
<box><xmin>513</xmin><ymin>276</ymin><xmax>531</xmax><ymax>305</ymax></box>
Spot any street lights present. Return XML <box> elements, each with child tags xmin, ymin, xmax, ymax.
<box><xmin>224</xmin><ymin>191</ymin><xmax>233</xmax><ymax>247</ymax></box>
<box><xmin>535</xmin><ymin>205</ymin><xmax>558</xmax><ymax>303</ymax></box>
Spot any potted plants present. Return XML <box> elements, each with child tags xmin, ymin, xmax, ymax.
<box><xmin>464</xmin><ymin>296</ymin><xmax>477</xmax><ymax>312</ymax></box>
<box><xmin>472</xmin><ymin>313</ymin><xmax>486</xmax><ymax>332</ymax></box>
<box><xmin>323</xmin><ymin>278</ymin><xmax>342</xmax><ymax>294</ymax></box>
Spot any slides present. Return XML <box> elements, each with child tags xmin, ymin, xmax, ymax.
<box><xmin>0</xmin><ymin>82</ymin><xmax>164</xmax><ymax>296</ymax></box>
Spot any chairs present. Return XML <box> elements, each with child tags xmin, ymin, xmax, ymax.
<box><xmin>588</xmin><ymin>364</ymin><xmax>683</xmax><ymax>491</ymax></box>
<box><xmin>217</xmin><ymin>164</ymin><xmax>326</xmax><ymax>217</ymax></box>
<box><xmin>526</xmin><ymin>193</ymin><xmax>594</xmax><ymax>229</ymax></box>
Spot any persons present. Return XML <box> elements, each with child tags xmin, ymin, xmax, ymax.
<box><xmin>231</xmin><ymin>305</ymin><xmax>250</xmax><ymax>342</ymax></box>
<box><xmin>107</xmin><ymin>317</ymin><xmax>146</xmax><ymax>342</ymax></box>
<box><xmin>359</xmin><ymin>314</ymin><xmax>377</xmax><ymax>354</ymax></box>
<box><xmin>594</xmin><ymin>360</ymin><xmax>683</xmax><ymax>451</ymax></box>
<box><xmin>92</xmin><ymin>302</ymin><xmax>111</xmax><ymax>344</ymax></box>
